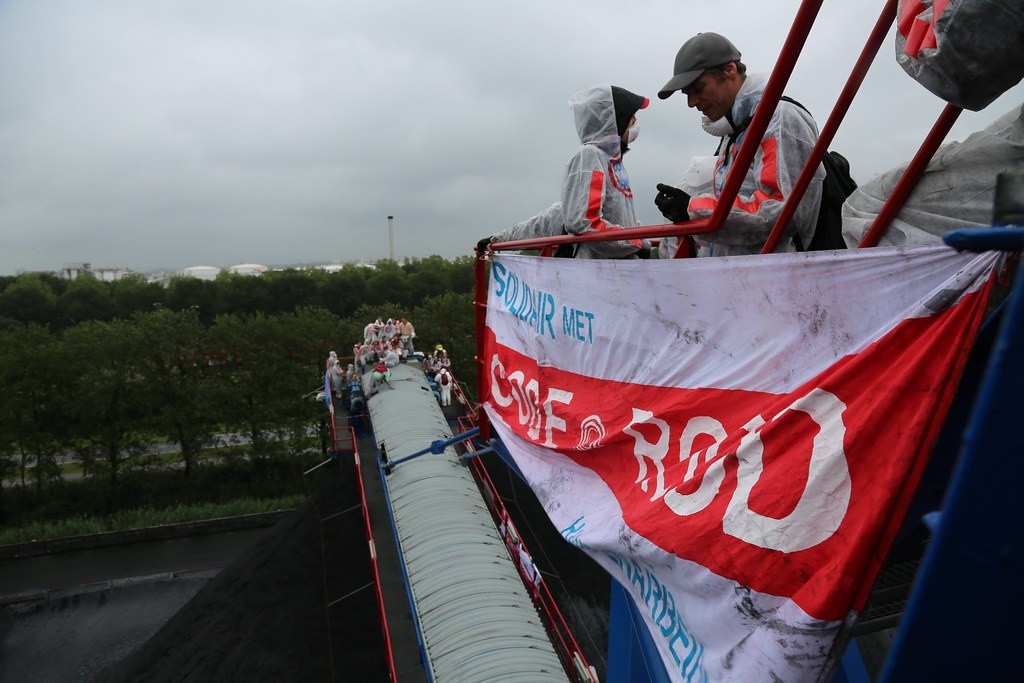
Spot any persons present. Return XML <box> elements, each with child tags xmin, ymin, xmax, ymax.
<box><xmin>654</xmin><ymin>32</ymin><xmax>827</xmax><ymax>260</ymax></box>
<box><xmin>326</xmin><ymin>316</ymin><xmax>453</xmax><ymax>408</ymax></box>
<box><xmin>476</xmin><ymin>198</ymin><xmax>574</xmax><ymax>259</ymax></box>
<box><xmin>560</xmin><ymin>86</ymin><xmax>653</xmax><ymax>259</ymax></box>
<box><xmin>841</xmin><ymin>0</ymin><xmax>1024</xmax><ymax>249</ymax></box>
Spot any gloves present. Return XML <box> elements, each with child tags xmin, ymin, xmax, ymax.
<box><xmin>653</xmin><ymin>183</ymin><xmax>691</xmax><ymax>223</ymax></box>
<box><xmin>477</xmin><ymin>236</ymin><xmax>493</xmax><ymax>253</ymax></box>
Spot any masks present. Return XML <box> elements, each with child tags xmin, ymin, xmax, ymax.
<box><xmin>625</xmin><ymin>119</ymin><xmax>639</xmax><ymax>144</ymax></box>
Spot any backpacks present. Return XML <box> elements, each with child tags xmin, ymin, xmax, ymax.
<box><xmin>723</xmin><ymin>95</ymin><xmax>858</xmax><ymax>250</ymax></box>
<box><xmin>436</xmin><ymin>350</ymin><xmax>443</xmax><ymax>358</ymax></box>
<box><xmin>440</xmin><ymin>373</ymin><xmax>449</xmax><ymax>385</ymax></box>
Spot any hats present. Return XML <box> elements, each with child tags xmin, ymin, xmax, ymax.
<box><xmin>658</xmin><ymin>32</ymin><xmax>741</xmax><ymax>99</ymax></box>
<box><xmin>611</xmin><ymin>86</ymin><xmax>650</xmax><ymax>137</ymax></box>
